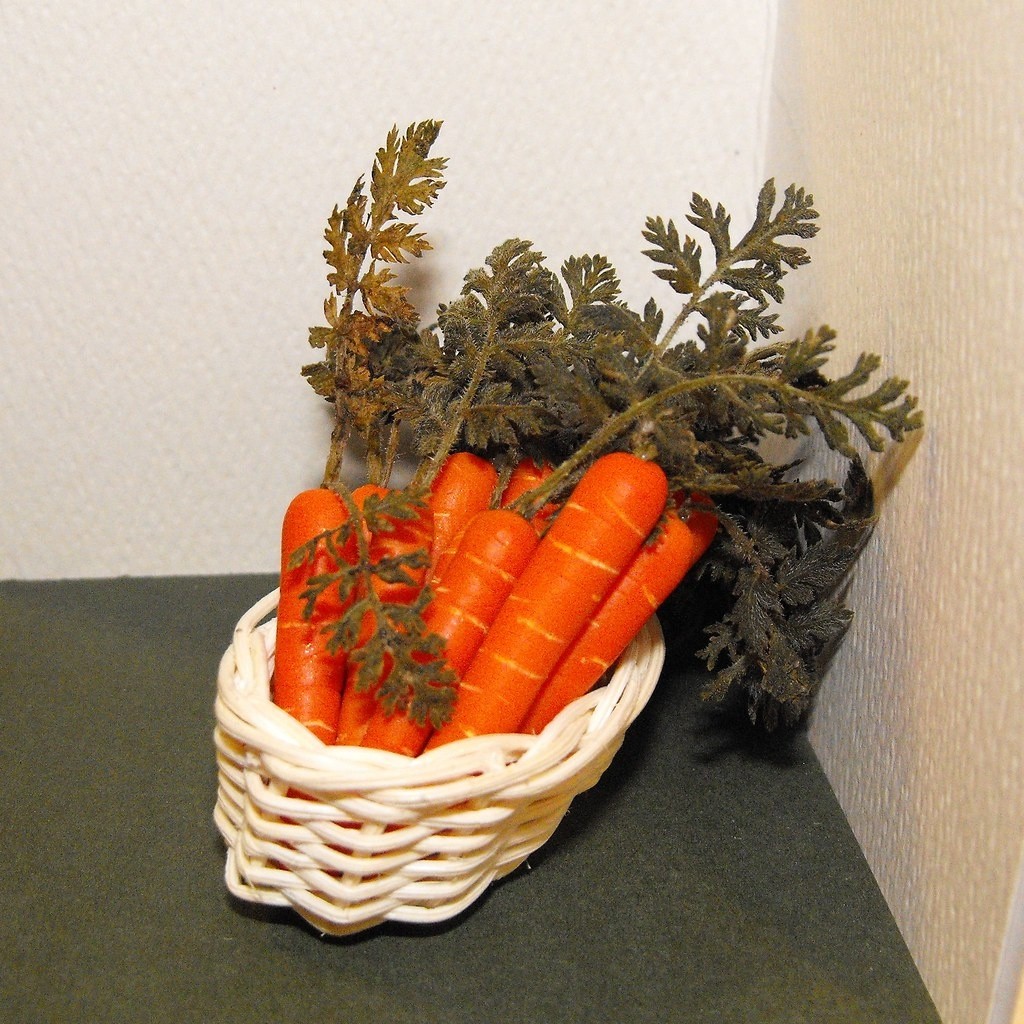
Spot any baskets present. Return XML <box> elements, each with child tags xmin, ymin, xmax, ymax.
<box><xmin>212</xmin><ymin>580</ymin><xmax>666</xmax><ymax>937</ymax></box>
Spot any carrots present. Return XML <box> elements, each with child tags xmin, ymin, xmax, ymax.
<box><xmin>267</xmin><ymin>451</ymin><xmax>720</xmax><ymax>879</ymax></box>
